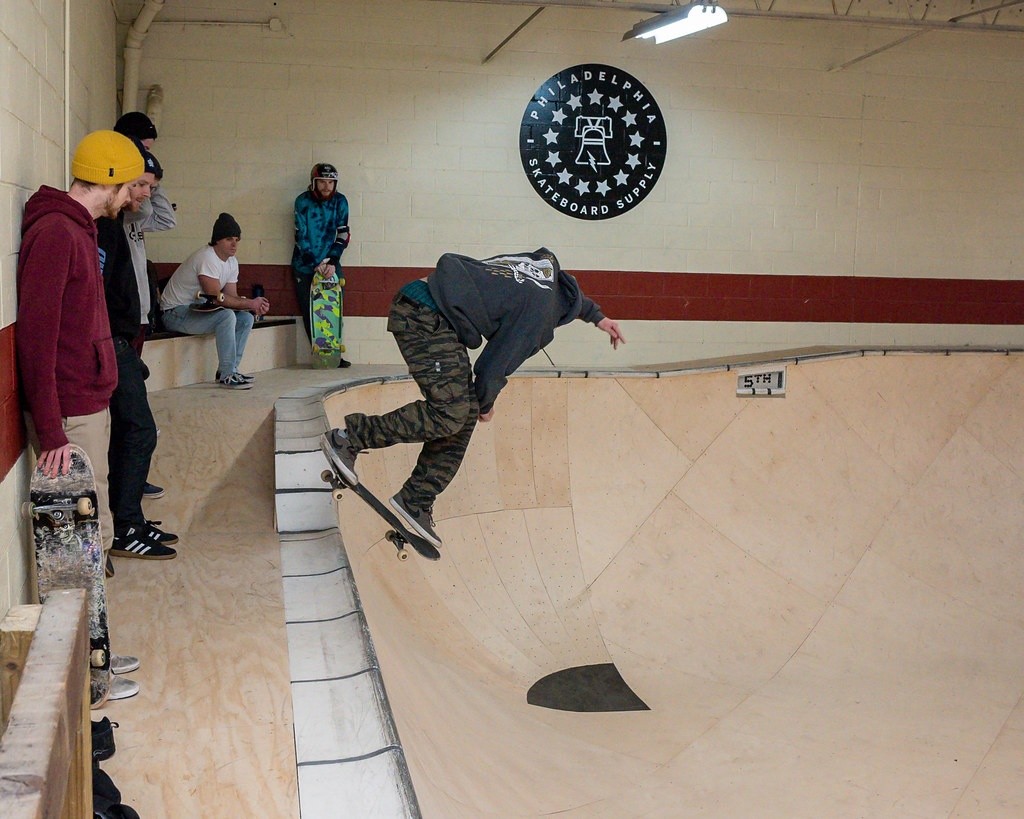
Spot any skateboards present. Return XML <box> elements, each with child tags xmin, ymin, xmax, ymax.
<box><xmin>319</xmin><ymin>442</ymin><xmax>441</xmax><ymax>562</ymax></box>
<box><xmin>189</xmin><ymin>290</ymin><xmax>255</xmax><ymax>312</ymax></box>
<box><xmin>310</xmin><ymin>270</ymin><xmax>345</xmax><ymax>369</ymax></box>
<box><xmin>19</xmin><ymin>442</ymin><xmax>112</xmax><ymax>710</ymax></box>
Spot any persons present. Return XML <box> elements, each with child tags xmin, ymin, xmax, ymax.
<box><xmin>322</xmin><ymin>247</ymin><xmax>626</xmax><ymax>549</ymax></box>
<box><xmin>15</xmin><ymin>130</ymin><xmax>145</xmax><ymax>699</ymax></box>
<box><xmin>290</xmin><ymin>163</ymin><xmax>352</xmax><ymax>368</ymax></box>
<box><xmin>95</xmin><ymin>112</ymin><xmax>180</xmax><ymax>560</ymax></box>
<box><xmin>160</xmin><ymin>213</ymin><xmax>269</xmax><ymax>390</ymax></box>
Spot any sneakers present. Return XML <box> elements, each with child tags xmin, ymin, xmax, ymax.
<box><xmin>216</xmin><ymin>371</ymin><xmax>255</xmax><ymax>384</ymax></box>
<box><xmin>389</xmin><ymin>489</ymin><xmax>442</xmax><ymax>548</ymax></box>
<box><xmin>320</xmin><ymin>428</ymin><xmax>370</xmax><ymax>486</ymax></box>
<box><xmin>110</xmin><ymin>527</ymin><xmax>177</xmax><ymax>560</ymax></box>
<box><xmin>219</xmin><ymin>374</ymin><xmax>253</xmax><ymax>390</ymax></box>
<box><xmin>144</xmin><ymin>521</ymin><xmax>179</xmax><ymax>545</ymax></box>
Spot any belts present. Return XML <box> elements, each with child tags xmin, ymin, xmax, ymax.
<box><xmin>117</xmin><ymin>335</ymin><xmax>130</xmax><ymax>345</ymax></box>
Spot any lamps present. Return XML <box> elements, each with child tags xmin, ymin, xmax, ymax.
<box><xmin>620</xmin><ymin>0</ymin><xmax>728</xmax><ymax>45</ymax></box>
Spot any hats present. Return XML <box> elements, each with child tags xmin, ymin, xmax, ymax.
<box><xmin>114</xmin><ymin>111</ymin><xmax>157</xmax><ymax>140</ymax></box>
<box><xmin>71</xmin><ymin>130</ymin><xmax>144</xmax><ymax>186</ymax></box>
<box><xmin>211</xmin><ymin>213</ymin><xmax>241</xmax><ymax>244</ymax></box>
<box><xmin>141</xmin><ymin>150</ymin><xmax>163</xmax><ymax>179</ymax></box>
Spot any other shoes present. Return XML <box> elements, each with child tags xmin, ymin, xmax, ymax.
<box><xmin>338</xmin><ymin>358</ymin><xmax>351</xmax><ymax>368</ymax></box>
<box><xmin>142</xmin><ymin>483</ymin><xmax>165</xmax><ymax>499</ymax></box>
<box><xmin>90</xmin><ymin>716</ymin><xmax>120</xmax><ymax>761</ymax></box>
<box><xmin>111</xmin><ymin>653</ymin><xmax>139</xmax><ymax>674</ymax></box>
<box><xmin>107</xmin><ymin>671</ymin><xmax>140</xmax><ymax>700</ymax></box>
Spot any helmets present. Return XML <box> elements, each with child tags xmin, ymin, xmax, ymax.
<box><xmin>310</xmin><ymin>163</ymin><xmax>338</xmax><ymax>192</ymax></box>
